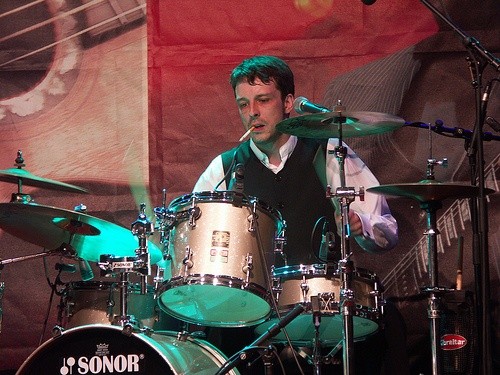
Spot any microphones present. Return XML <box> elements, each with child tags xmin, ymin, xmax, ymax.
<box><xmin>467</xmin><ymin>81</ymin><xmax>495</xmax><ymax>156</ymax></box>
<box><xmin>77</xmin><ymin>258</ymin><xmax>94</xmax><ymax>281</ymax></box>
<box><xmin>228</xmin><ymin>161</ymin><xmax>237</xmax><ymax>191</ymax></box>
<box><xmin>292</xmin><ymin>96</ymin><xmax>332</xmax><ymax>114</ymax></box>
<box><xmin>319</xmin><ymin>221</ymin><xmax>331</xmax><ymax>261</ymax></box>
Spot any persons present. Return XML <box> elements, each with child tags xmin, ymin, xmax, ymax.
<box><xmin>194</xmin><ymin>55</ymin><xmax>399</xmax><ymax>375</ymax></box>
<box><xmin>320</xmin><ymin>0</ymin><xmax>500</xmax><ymax>300</ymax></box>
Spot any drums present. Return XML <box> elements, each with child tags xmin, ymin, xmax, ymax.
<box><xmin>65</xmin><ymin>280</ymin><xmax>180</xmax><ymax>333</ymax></box>
<box><xmin>14</xmin><ymin>323</ymin><xmax>241</xmax><ymax>375</ymax></box>
<box><xmin>152</xmin><ymin>189</ymin><xmax>282</xmax><ymax>328</ymax></box>
<box><xmin>250</xmin><ymin>262</ymin><xmax>383</xmax><ymax>344</ymax></box>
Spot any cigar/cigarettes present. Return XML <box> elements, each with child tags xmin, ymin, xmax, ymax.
<box><xmin>239</xmin><ymin>126</ymin><xmax>255</xmax><ymax>141</ymax></box>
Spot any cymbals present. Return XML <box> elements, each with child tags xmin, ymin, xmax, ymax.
<box><xmin>0</xmin><ymin>202</ymin><xmax>164</xmax><ymax>266</ymax></box>
<box><xmin>0</xmin><ymin>167</ymin><xmax>91</xmax><ymax>195</ymax></box>
<box><xmin>365</xmin><ymin>179</ymin><xmax>496</xmax><ymax>201</ymax></box>
<box><xmin>274</xmin><ymin>110</ymin><xmax>406</xmax><ymax>138</ymax></box>
